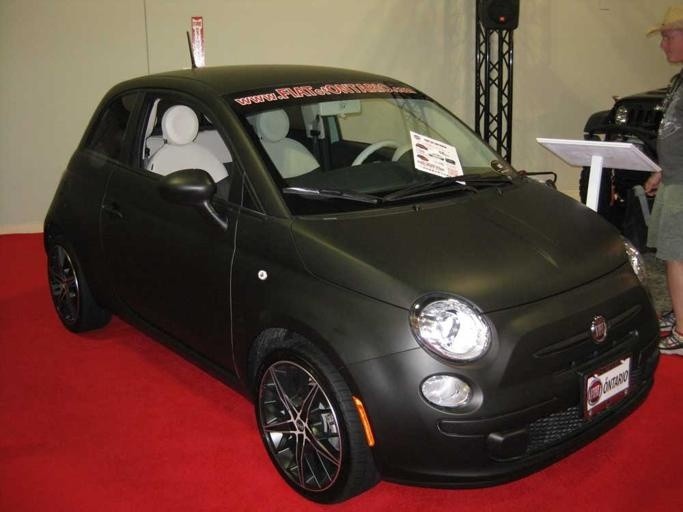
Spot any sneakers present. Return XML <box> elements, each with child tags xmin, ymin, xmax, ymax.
<box><xmin>658</xmin><ymin>326</ymin><xmax>683</xmax><ymax>356</ymax></box>
<box><xmin>658</xmin><ymin>310</ymin><xmax>676</xmax><ymax>331</ymax></box>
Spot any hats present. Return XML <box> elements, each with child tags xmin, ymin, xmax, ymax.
<box><xmin>646</xmin><ymin>7</ymin><xmax>683</xmax><ymax>39</ymax></box>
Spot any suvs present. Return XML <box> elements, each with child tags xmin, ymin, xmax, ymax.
<box><xmin>573</xmin><ymin>84</ymin><xmax>668</xmax><ymax>219</ymax></box>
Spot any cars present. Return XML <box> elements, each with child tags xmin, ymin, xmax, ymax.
<box><xmin>41</xmin><ymin>29</ymin><xmax>661</xmax><ymax>506</ymax></box>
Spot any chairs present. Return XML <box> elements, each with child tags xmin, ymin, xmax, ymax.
<box><xmin>146</xmin><ymin>104</ymin><xmax>229</xmax><ymax>184</ymax></box>
<box><xmin>252</xmin><ymin>109</ymin><xmax>320</xmax><ymax>181</ymax></box>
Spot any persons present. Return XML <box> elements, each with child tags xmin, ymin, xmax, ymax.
<box><xmin>644</xmin><ymin>5</ymin><xmax>683</xmax><ymax>356</ymax></box>
